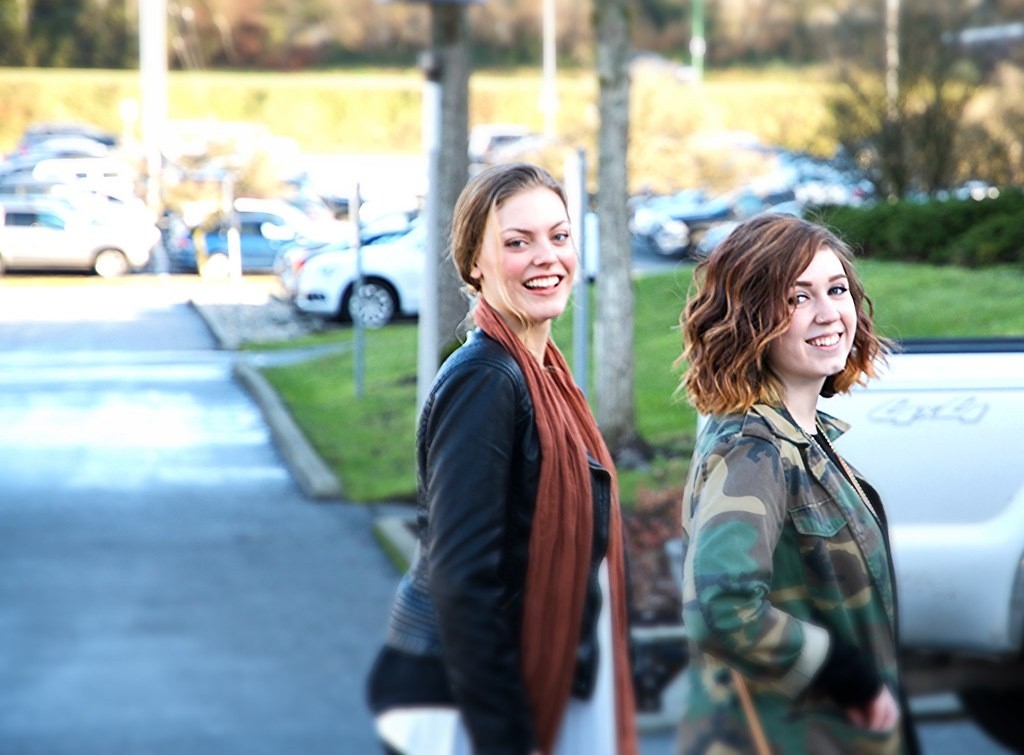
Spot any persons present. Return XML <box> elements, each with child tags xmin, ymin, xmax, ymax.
<box><xmin>356</xmin><ymin>158</ymin><xmax>644</xmax><ymax>755</ymax></box>
<box><xmin>666</xmin><ymin>211</ymin><xmax>921</xmax><ymax>754</ymax></box>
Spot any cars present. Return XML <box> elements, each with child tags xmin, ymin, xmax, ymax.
<box><xmin>2</xmin><ymin>124</ymin><xmax>1004</xmax><ymax>334</ymax></box>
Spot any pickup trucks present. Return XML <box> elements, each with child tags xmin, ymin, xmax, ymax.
<box><xmin>692</xmin><ymin>331</ymin><xmax>1024</xmax><ymax>755</ymax></box>
